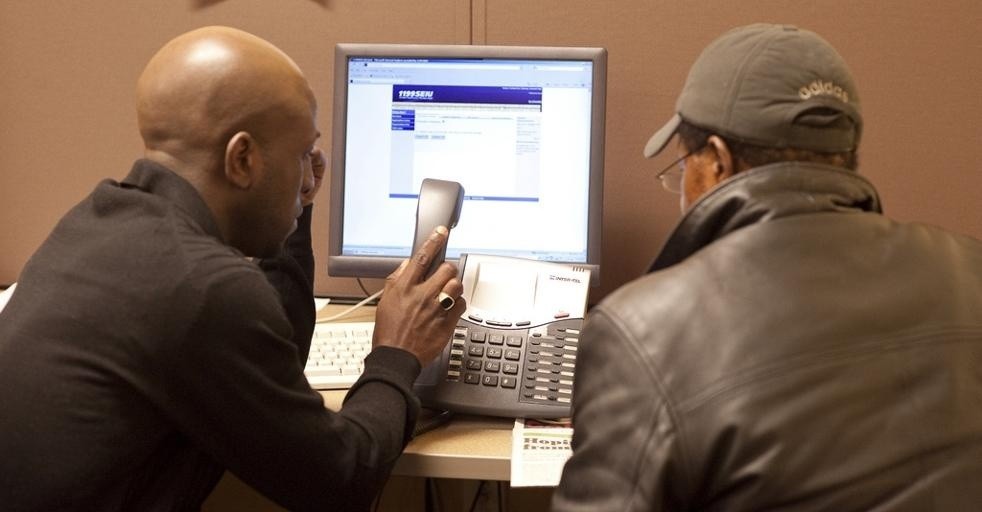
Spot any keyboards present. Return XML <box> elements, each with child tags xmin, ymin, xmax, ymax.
<box><xmin>302</xmin><ymin>320</ymin><xmax>376</xmax><ymax>388</ymax></box>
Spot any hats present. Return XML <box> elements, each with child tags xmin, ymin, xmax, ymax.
<box><xmin>639</xmin><ymin>20</ymin><xmax>866</xmax><ymax>162</ymax></box>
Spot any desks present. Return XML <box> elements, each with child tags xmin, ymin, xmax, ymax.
<box><xmin>305</xmin><ymin>304</ymin><xmax>572</xmax><ymax>512</ymax></box>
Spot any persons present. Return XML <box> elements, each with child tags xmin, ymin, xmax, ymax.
<box><xmin>550</xmin><ymin>22</ymin><xmax>982</xmax><ymax>511</ymax></box>
<box><xmin>1</xmin><ymin>24</ymin><xmax>466</xmax><ymax>511</ymax></box>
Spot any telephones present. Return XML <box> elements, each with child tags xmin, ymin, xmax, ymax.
<box><xmin>409</xmin><ymin>178</ymin><xmax>602</xmax><ymax>418</ymax></box>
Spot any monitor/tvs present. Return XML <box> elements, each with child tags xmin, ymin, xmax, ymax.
<box><xmin>327</xmin><ymin>40</ymin><xmax>609</xmax><ymax>284</ymax></box>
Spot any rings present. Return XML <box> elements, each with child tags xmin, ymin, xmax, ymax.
<box><xmin>438</xmin><ymin>292</ymin><xmax>455</xmax><ymax>311</ymax></box>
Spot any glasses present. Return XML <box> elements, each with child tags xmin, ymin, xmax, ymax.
<box><xmin>654</xmin><ymin>141</ymin><xmax>712</xmax><ymax>194</ymax></box>
